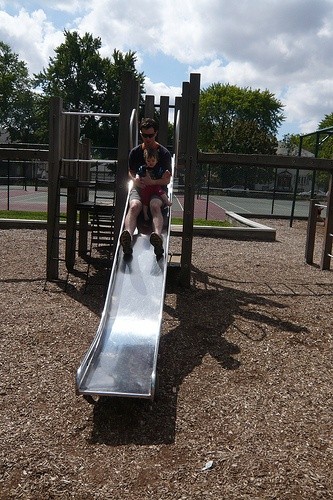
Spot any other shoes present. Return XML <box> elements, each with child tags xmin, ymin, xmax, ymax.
<box><xmin>149</xmin><ymin>232</ymin><xmax>164</xmax><ymax>255</ymax></box>
<box><xmin>120</xmin><ymin>230</ymin><xmax>132</xmax><ymax>254</ymax></box>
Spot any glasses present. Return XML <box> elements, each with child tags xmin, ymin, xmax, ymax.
<box><xmin>140</xmin><ymin>130</ymin><xmax>155</xmax><ymax>138</ymax></box>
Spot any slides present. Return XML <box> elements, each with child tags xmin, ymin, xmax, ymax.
<box><xmin>78</xmin><ymin>151</ymin><xmax>174</xmax><ymax>399</ymax></box>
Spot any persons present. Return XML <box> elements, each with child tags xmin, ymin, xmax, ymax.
<box><xmin>133</xmin><ymin>146</ymin><xmax>172</xmax><ymax>222</ymax></box>
<box><xmin>119</xmin><ymin>117</ymin><xmax>172</xmax><ymax>257</ymax></box>
